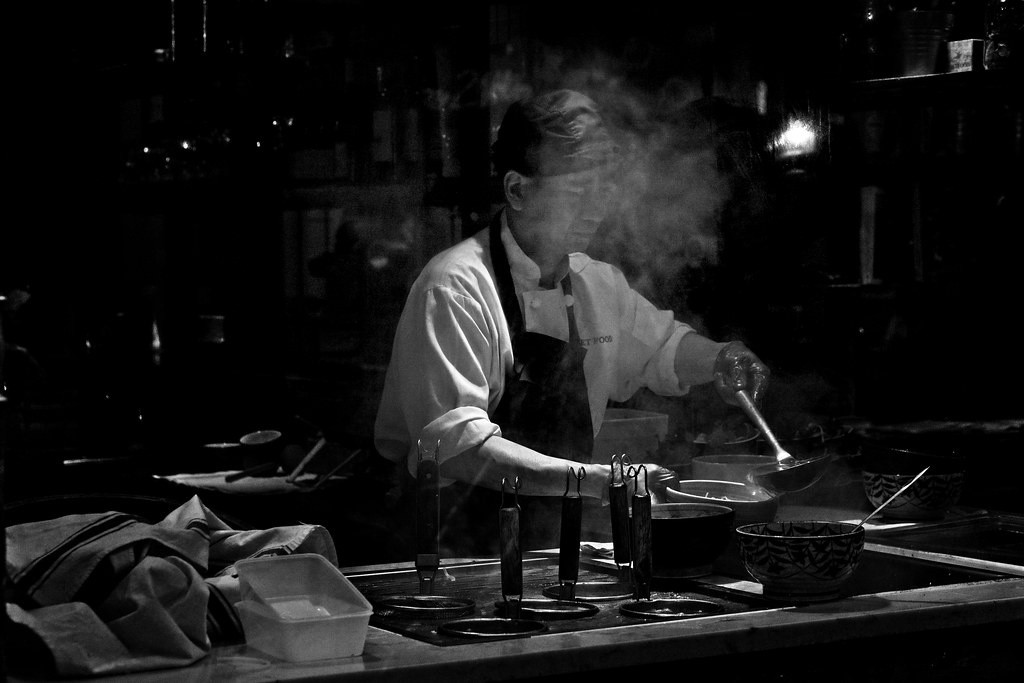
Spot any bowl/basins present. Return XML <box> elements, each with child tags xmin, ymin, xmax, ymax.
<box><xmin>860</xmin><ymin>469</ymin><xmax>969</xmax><ymax>521</ymax></box>
<box><xmin>626</xmin><ymin>502</ymin><xmax>736</xmax><ymax>568</ymax></box>
<box><xmin>692</xmin><ymin>454</ymin><xmax>777</xmax><ymax>484</ymax></box>
<box><xmin>666</xmin><ymin>479</ymin><xmax>780</xmax><ymax>524</ymax></box>
<box><xmin>736</xmin><ymin>520</ymin><xmax>865</xmax><ymax>595</ymax></box>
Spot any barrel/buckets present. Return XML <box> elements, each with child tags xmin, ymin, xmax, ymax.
<box><xmin>893</xmin><ymin>10</ymin><xmax>953</xmax><ymax>74</ymax></box>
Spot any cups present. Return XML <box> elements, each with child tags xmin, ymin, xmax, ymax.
<box><xmin>205</xmin><ymin>442</ymin><xmax>241</xmax><ymax>473</ymax></box>
<box><xmin>239</xmin><ymin>430</ymin><xmax>283</xmax><ymax>476</ymax></box>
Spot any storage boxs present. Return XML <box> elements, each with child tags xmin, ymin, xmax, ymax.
<box><xmin>235</xmin><ymin>553</ymin><xmax>374</xmax><ymax>663</ymax></box>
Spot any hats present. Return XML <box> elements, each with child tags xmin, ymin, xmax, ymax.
<box><xmin>490</xmin><ymin>89</ymin><xmax>624</xmax><ymax>179</ymax></box>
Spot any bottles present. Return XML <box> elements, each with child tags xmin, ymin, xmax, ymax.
<box><xmin>290</xmin><ymin>55</ymin><xmax>425</xmax><ymax>186</ymax></box>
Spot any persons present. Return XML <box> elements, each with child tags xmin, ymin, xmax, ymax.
<box><xmin>374</xmin><ymin>89</ymin><xmax>771</xmax><ymax>560</ymax></box>
